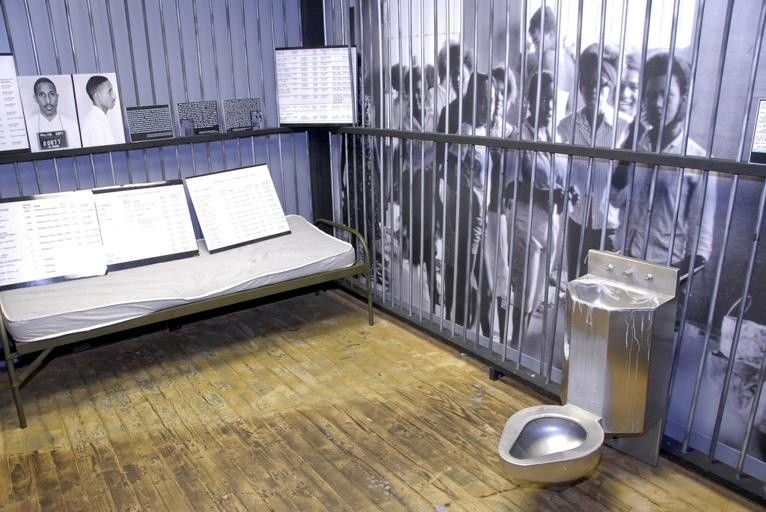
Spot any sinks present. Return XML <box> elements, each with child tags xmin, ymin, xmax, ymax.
<box><xmin>574</xmin><ymin>282</ymin><xmax>659</xmax><ymax>310</ymax></box>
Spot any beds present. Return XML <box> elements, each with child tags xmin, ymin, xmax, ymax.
<box><xmin>0</xmin><ymin>212</ymin><xmax>374</xmax><ymax>431</ymax></box>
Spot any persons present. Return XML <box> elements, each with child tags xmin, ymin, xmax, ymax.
<box><xmin>81</xmin><ymin>77</ymin><xmax>122</xmax><ymax>147</ymax></box>
<box><xmin>337</xmin><ymin>5</ymin><xmax>721</xmax><ymax>355</ymax></box>
<box><xmin>29</xmin><ymin>79</ymin><xmax>80</xmax><ymax>151</ymax></box>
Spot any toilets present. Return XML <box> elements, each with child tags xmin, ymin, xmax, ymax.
<box><xmin>498</xmin><ymin>248</ymin><xmax>681</xmax><ymax>490</ymax></box>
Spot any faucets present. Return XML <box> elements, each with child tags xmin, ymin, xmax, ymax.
<box><xmin>620</xmin><ymin>268</ymin><xmax>633</xmax><ymax>279</ymax></box>
